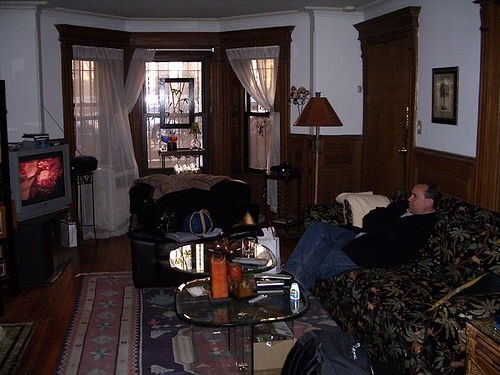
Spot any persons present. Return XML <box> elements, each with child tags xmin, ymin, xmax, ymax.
<box><xmin>285</xmin><ymin>183</ymin><xmax>442</xmax><ymax>295</ymax></box>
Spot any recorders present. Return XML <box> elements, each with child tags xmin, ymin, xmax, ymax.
<box><xmin>42</xmin><ymin>107</ymin><xmax>98</xmax><ymax>174</ymax></box>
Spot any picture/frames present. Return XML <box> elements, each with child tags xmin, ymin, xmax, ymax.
<box><xmin>431</xmin><ymin>66</ymin><xmax>459</xmax><ymax>126</ymax></box>
<box><xmin>160</xmin><ymin>78</ymin><xmax>194</xmax><ymax>129</ymax></box>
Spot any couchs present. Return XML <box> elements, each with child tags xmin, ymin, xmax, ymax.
<box><xmin>129</xmin><ymin>174</ymin><xmax>261</xmax><ymax>288</ymax></box>
<box><xmin>305</xmin><ymin>192</ymin><xmax>500</xmax><ymax>375</ymax></box>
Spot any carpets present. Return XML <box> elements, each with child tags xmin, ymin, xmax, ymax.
<box><xmin>53</xmin><ymin>269</ymin><xmax>343</xmax><ymax>375</ymax></box>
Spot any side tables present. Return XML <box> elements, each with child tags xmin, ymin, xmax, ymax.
<box><xmin>465</xmin><ymin>318</ymin><xmax>500</xmax><ymax>375</ymax></box>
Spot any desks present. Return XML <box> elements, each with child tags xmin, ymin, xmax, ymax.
<box><xmin>158</xmin><ymin>147</ymin><xmax>205</xmax><ymax>169</ymax></box>
<box><xmin>168</xmin><ymin>237</ymin><xmax>309</xmax><ymax>375</ymax></box>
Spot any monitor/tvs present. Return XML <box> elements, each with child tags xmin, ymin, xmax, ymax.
<box><xmin>8</xmin><ymin>143</ymin><xmax>72</xmax><ymax>222</ymax></box>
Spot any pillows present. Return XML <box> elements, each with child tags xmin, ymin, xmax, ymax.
<box><xmin>415</xmin><ymin>219</ymin><xmax>491</xmax><ymax>281</ymax></box>
<box><xmin>335</xmin><ymin>190</ymin><xmax>391</xmax><ymax>228</ymax></box>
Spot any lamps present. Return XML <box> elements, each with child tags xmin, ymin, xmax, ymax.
<box><xmin>293</xmin><ymin>92</ymin><xmax>343</xmax><ymax>205</ymax></box>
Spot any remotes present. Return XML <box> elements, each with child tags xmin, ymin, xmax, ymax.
<box><xmin>257</xmin><ymin>280</ymin><xmax>284</xmax><ymax>286</ymax></box>
<box><xmin>232</xmin><ymin>257</ymin><xmax>268</xmax><ymax>266</ymax></box>
<box><xmin>254</xmin><ymin>273</ymin><xmax>292</xmax><ymax>280</ymax></box>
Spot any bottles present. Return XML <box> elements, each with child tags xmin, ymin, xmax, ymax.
<box><xmin>288</xmin><ymin>282</ymin><xmax>300</xmax><ymax>299</ymax></box>
<box><xmin>210</xmin><ymin>249</ymin><xmax>228</xmax><ymax>298</ymax></box>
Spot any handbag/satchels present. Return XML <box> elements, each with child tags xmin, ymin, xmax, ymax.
<box><xmin>281</xmin><ymin>324</ymin><xmax>376</xmax><ymax>375</ymax></box>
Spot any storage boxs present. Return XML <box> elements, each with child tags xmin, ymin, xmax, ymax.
<box><xmin>253</xmin><ymin>321</ymin><xmax>298</xmax><ymax>370</ymax></box>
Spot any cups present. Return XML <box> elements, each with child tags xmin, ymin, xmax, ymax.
<box><xmin>242</xmin><ymin>237</ymin><xmax>255</xmax><ymax>256</ymax></box>
